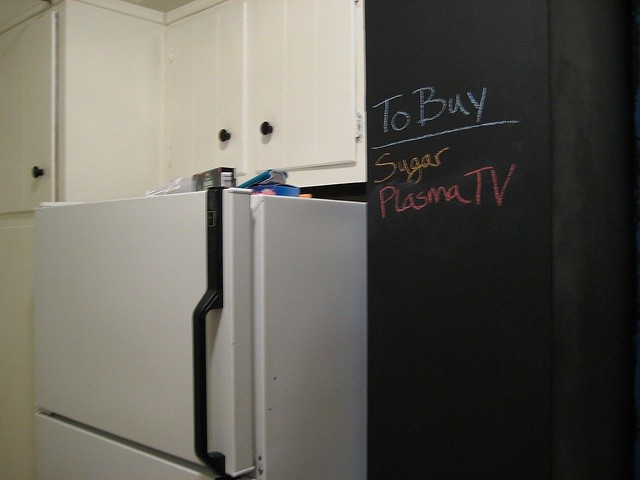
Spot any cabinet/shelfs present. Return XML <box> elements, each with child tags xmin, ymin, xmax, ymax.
<box><xmin>0</xmin><ymin>2</ymin><xmax>163</xmax><ymax>217</ymax></box>
<box><xmin>0</xmin><ymin>218</ymin><xmax>38</xmax><ymax>480</ymax></box>
<box><xmin>164</xmin><ymin>0</ymin><xmax>367</xmax><ymax>188</ymax></box>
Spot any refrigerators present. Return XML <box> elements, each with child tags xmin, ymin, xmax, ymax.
<box><xmin>34</xmin><ymin>188</ymin><xmax>368</xmax><ymax>480</ymax></box>
